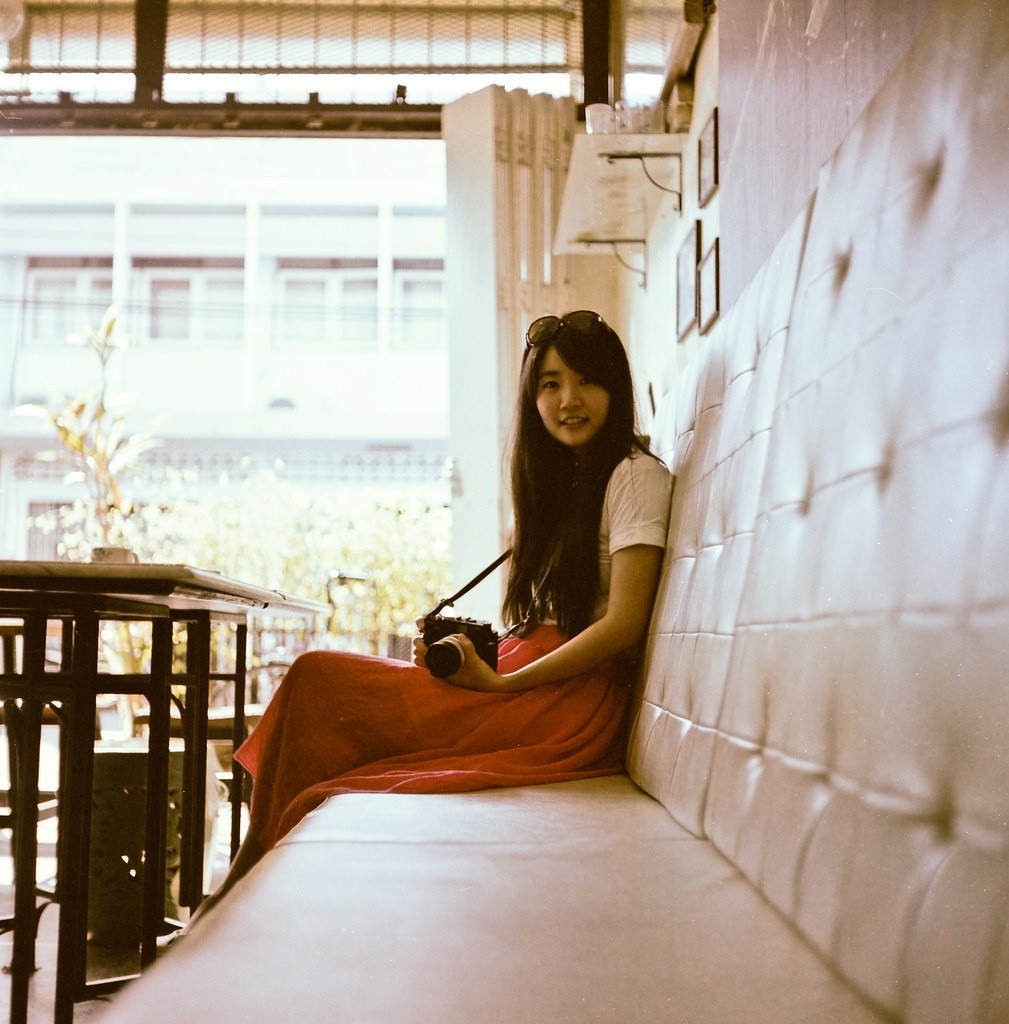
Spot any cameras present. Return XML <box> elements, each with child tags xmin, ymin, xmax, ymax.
<box><xmin>422</xmin><ymin>617</ymin><xmax>498</xmax><ymax>677</ymax></box>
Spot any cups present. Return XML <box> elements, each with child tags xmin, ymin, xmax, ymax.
<box><xmin>91</xmin><ymin>548</ymin><xmax>139</xmax><ymax>564</ymax></box>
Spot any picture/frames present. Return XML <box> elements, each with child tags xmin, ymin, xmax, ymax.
<box><xmin>675</xmin><ymin>218</ymin><xmax>701</xmax><ymax>344</ymax></box>
<box><xmin>695</xmin><ymin>236</ymin><xmax>720</xmax><ymax>336</ymax></box>
<box><xmin>695</xmin><ymin>104</ymin><xmax>719</xmax><ymax>211</ymax></box>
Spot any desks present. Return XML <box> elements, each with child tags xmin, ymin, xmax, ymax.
<box><xmin>0</xmin><ymin>559</ymin><xmax>333</xmax><ymax>1024</ymax></box>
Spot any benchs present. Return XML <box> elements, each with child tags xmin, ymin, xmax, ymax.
<box><xmin>88</xmin><ymin>0</ymin><xmax>1009</xmax><ymax>1024</ymax></box>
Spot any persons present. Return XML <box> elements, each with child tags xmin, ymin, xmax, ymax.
<box><xmin>139</xmin><ymin>309</ymin><xmax>674</xmax><ymax>954</ymax></box>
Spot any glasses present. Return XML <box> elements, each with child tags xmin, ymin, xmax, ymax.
<box><xmin>526</xmin><ymin>310</ymin><xmax>611</xmax><ymax>355</ymax></box>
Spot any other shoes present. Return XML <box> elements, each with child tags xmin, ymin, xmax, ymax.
<box><xmin>140</xmin><ymin>930</ymin><xmax>184</xmax><ymax>955</ymax></box>
